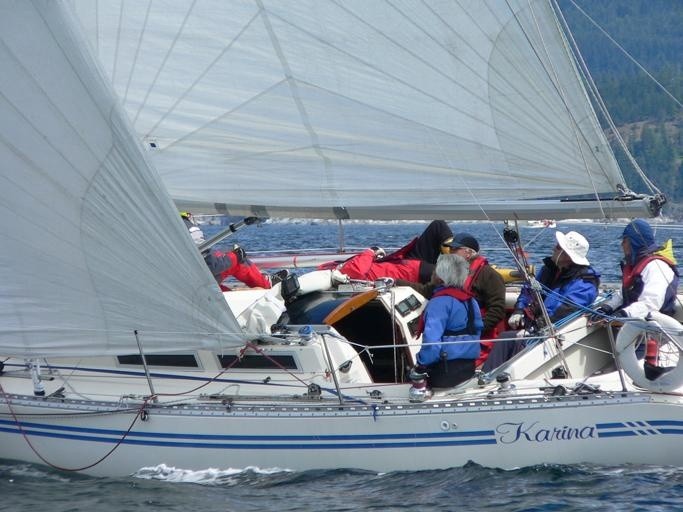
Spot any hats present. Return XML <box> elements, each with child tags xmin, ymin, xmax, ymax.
<box><xmin>442</xmin><ymin>233</ymin><xmax>479</xmax><ymax>252</ymax></box>
<box><xmin>555</xmin><ymin>229</ymin><xmax>591</xmax><ymax>266</ymax></box>
<box><xmin>617</xmin><ymin>220</ymin><xmax>654</xmax><ymax>247</ymax></box>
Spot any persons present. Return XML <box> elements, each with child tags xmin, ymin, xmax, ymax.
<box><xmin>317</xmin><ymin>218</ymin><xmax>505</xmax><ymax>388</ymax></box>
<box><xmin>179</xmin><ymin>212</ymin><xmax>290</xmax><ymax>291</ymax></box>
<box><xmin>481</xmin><ymin>231</ymin><xmax>601</xmax><ymax>373</ymax></box>
<box><xmin>582</xmin><ymin>219</ymin><xmax>678</xmax><ymax>382</ymax></box>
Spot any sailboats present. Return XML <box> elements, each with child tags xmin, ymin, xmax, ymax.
<box><xmin>2</xmin><ymin>0</ymin><xmax>682</xmax><ymax>480</ymax></box>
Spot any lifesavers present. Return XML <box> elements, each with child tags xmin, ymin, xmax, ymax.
<box><xmin>616</xmin><ymin>310</ymin><xmax>683</xmax><ymax>394</ymax></box>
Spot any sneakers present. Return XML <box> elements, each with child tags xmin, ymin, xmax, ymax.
<box><xmin>271</xmin><ymin>269</ymin><xmax>289</xmax><ymax>287</ymax></box>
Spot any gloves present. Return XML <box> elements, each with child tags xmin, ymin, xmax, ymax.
<box><xmin>516</xmin><ymin>329</ymin><xmax>530</xmax><ymax>338</ymax></box>
<box><xmin>233</xmin><ymin>246</ymin><xmax>246</xmax><ymax>264</ymax></box>
<box><xmin>612</xmin><ymin>310</ymin><xmax>627</xmax><ymax>318</ymax></box>
<box><xmin>509</xmin><ymin>314</ymin><xmax>524</xmax><ymax>329</ymax></box>
<box><xmin>589</xmin><ymin>304</ymin><xmax>613</xmax><ymax>322</ymax></box>
